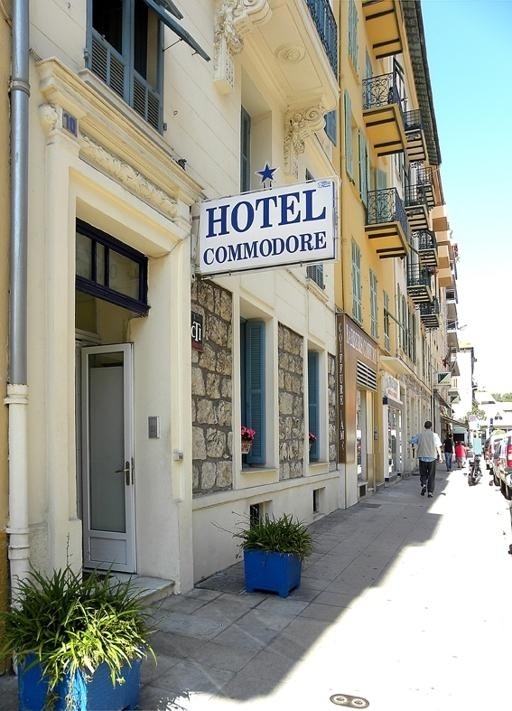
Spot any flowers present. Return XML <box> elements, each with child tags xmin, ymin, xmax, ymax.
<box><xmin>309</xmin><ymin>432</ymin><xmax>316</xmax><ymax>441</ymax></box>
<box><xmin>241</xmin><ymin>425</ymin><xmax>256</xmax><ymax>441</ymax></box>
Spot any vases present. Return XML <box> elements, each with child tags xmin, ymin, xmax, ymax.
<box><xmin>310</xmin><ymin>443</ymin><xmax>312</xmax><ymax>451</ymax></box>
<box><xmin>242</xmin><ymin>442</ymin><xmax>252</xmax><ymax>454</ymax></box>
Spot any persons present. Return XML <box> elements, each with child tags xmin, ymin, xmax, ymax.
<box><xmin>407</xmin><ymin>419</ymin><xmax>445</xmax><ymax>498</ymax></box>
<box><xmin>454</xmin><ymin>440</ymin><xmax>467</xmax><ymax>469</ymax></box>
<box><xmin>441</xmin><ymin>432</ymin><xmax>455</xmax><ymax>472</ymax></box>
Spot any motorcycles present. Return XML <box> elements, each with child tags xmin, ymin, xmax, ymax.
<box><xmin>468</xmin><ymin>457</ymin><xmax>483</xmax><ymax>486</ymax></box>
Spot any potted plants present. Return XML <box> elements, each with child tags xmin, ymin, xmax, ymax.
<box><xmin>210</xmin><ymin>505</ymin><xmax>321</xmax><ymax>598</ymax></box>
<box><xmin>0</xmin><ymin>556</ymin><xmax>170</xmax><ymax>711</ymax></box>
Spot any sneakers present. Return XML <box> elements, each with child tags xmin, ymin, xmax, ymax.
<box><xmin>420</xmin><ymin>484</ymin><xmax>434</xmax><ymax>498</ymax></box>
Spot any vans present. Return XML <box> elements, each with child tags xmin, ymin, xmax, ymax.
<box><xmin>485</xmin><ymin>430</ymin><xmax>512</xmax><ymax>499</ymax></box>
